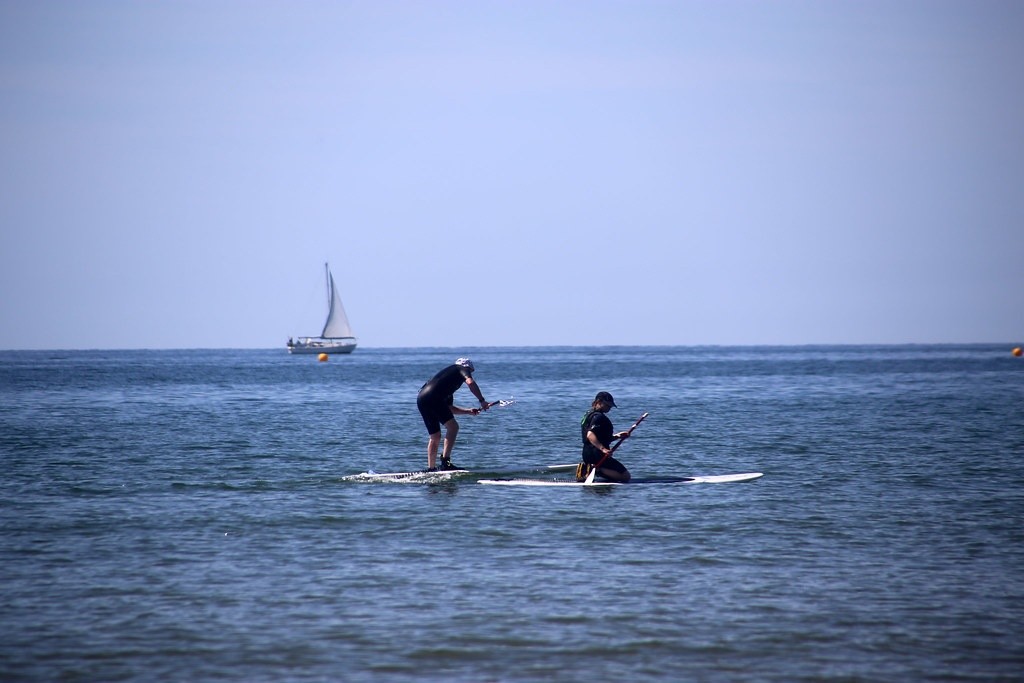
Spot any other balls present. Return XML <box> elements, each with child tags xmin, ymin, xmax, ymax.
<box><xmin>1013</xmin><ymin>348</ymin><xmax>1022</xmax><ymax>356</ymax></box>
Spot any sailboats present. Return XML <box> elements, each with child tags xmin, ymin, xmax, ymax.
<box><xmin>285</xmin><ymin>262</ymin><xmax>359</xmax><ymax>356</ymax></box>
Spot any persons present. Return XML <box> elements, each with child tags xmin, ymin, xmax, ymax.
<box><xmin>417</xmin><ymin>357</ymin><xmax>490</xmax><ymax>471</ymax></box>
<box><xmin>576</xmin><ymin>391</ymin><xmax>631</xmax><ymax>483</ymax></box>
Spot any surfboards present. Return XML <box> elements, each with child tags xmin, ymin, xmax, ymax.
<box><xmin>476</xmin><ymin>471</ymin><xmax>763</xmax><ymax>486</ymax></box>
<box><xmin>349</xmin><ymin>462</ymin><xmax>580</xmax><ymax>477</ymax></box>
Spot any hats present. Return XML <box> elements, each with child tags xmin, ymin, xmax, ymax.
<box><xmin>454</xmin><ymin>358</ymin><xmax>475</xmax><ymax>371</ymax></box>
<box><xmin>597</xmin><ymin>392</ymin><xmax>617</xmax><ymax>408</ymax></box>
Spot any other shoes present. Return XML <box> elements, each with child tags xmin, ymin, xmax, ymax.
<box><xmin>440</xmin><ymin>461</ymin><xmax>462</xmax><ymax>471</ymax></box>
<box><xmin>576</xmin><ymin>463</ymin><xmax>586</xmax><ymax>479</ymax></box>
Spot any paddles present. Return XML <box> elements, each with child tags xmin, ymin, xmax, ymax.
<box><xmin>473</xmin><ymin>399</ymin><xmax>513</xmax><ymax>411</ymax></box>
<box><xmin>584</xmin><ymin>411</ymin><xmax>649</xmax><ymax>484</ymax></box>
